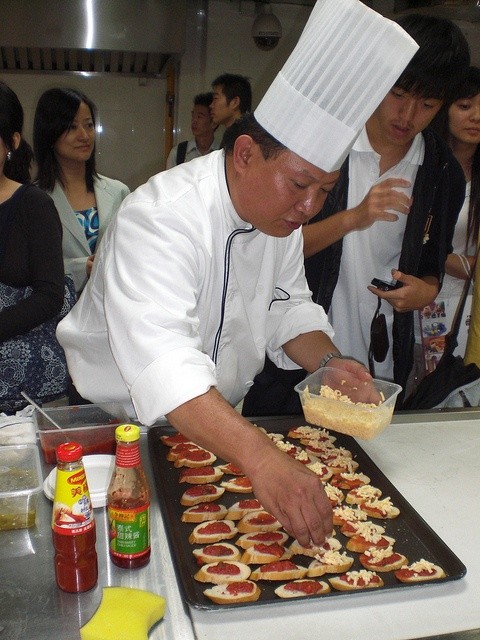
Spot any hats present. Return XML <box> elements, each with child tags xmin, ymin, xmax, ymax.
<box><xmin>255</xmin><ymin>1</ymin><xmax>420</xmax><ymax>174</ymax></box>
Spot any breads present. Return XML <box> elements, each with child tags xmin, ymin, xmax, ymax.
<box><xmin>226</xmin><ymin>497</ymin><xmax>263</xmax><ymax>521</ymax></box>
<box><xmin>307</xmin><ymin>551</ymin><xmax>355</xmax><ymax>578</ymax></box>
<box><xmin>193</xmin><ymin>560</ymin><xmax>252</xmax><ymax>584</ymax></box>
<box><xmin>287</xmin><ymin>450</ymin><xmax>311</xmax><ymax>464</ymax></box>
<box><xmin>347</xmin><ymin>532</ymin><xmax>390</xmax><ymax>552</ymax></box>
<box><xmin>290</xmin><ymin>535</ymin><xmax>343</xmax><ymax>556</ymax></box>
<box><xmin>275</xmin><ymin>579</ymin><xmax>331</xmax><ymax>597</ymax></box>
<box><xmin>330</xmin><ymin>472</ymin><xmax>370</xmax><ymax>489</ymax></box>
<box><xmin>266</xmin><ymin>432</ymin><xmax>284</xmax><ymax>442</ymax></box>
<box><xmin>330</xmin><ymin>569</ymin><xmax>384</xmax><ymax>590</ymax></box>
<box><xmin>182</xmin><ymin>502</ymin><xmax>229</xmax><ymax>523</ymax></box>
<box><xmin>302</xmin><ymin>430</ymin><xmax>337</xmax><ymax>445</ymax></box>
<box><xmin>345</xmin><ymin>486</ymin><xmax>382</xmax><ymax>505</ymax></box>
<box><xmin>393</xmin><ymin>558</ymin><xmax>447</xmax><ymax>584</ymax></box>
<box><xmin>320</xmin><ymin>446</ymin><xmax>352</xmax><ymax>460</ymax></box>
<box><xmin>235</xmin><ymin>508</ymin><xmax>284</xmax><ymax>532</ymax></box>
<box><xmin>304</xmin><ymin>439</ymin><xmax>333</xmax><ymax>456</ymax></box>
<box><xmin>326</xmin><ymin>457</ymin><xmax>360</xmax><ymax>473</ymax></box>
<box><xmin>219</xmin><ymin>475</ymin><xmax>253</xmax><ymax>493</ymax></box>
<box><xmin>181</xmin><ymin>482</ymin><xmax>226</xmax><ymax>507</ymax></box>
<box><xmin>240</xmin><ymin>541</ymin><xmax>293</xmax><ymax>564</ymax></box>
<box><xmin>179</xmin><ymin>464</ymin><xmax>224</xmax><ymax>485</ymax></box>
<box><xmin>286</xmin><ymin>425</ymin><xmax>312</xmax><ymax>437</ymax></box>
<box><xmin>341</xmin><ymin>519</ymin><xmax>381</xmax><ymax>538</ymax></box>
<box><xmin>217</xmin><ymin>462</ymin><xmax>245</xmax><ymax>476</ymax></box>
<box><xmin>189</xmin><ymin>518</ymin><xmax>238</xmax><ymax>545</ymax></box>
<box><xmin>235</xmin><ymin>527</ymin><xmax>289</xmax><ymax>548</ymax></box>
<box><xmin>167</xmin><ymin>442</ymin><xmax>199</xmax><ymax>462</ymax></box>
<box><xmin>202</xmin><ymin>578</ymin><xmax>262</xmax><ymax>605</ymax></box>
<box><xmin>191</xmin><ymin>542</ymin><xmax>241</xmax><ymax>563</ymax></box>
<box><xmin>361</xmin><ymin>496</ymin><xmax>400</xmax><ymax>519</ymax></box>
<box><xmin>360</xmin><ymin>546</ymin><xmax>409</xmax><ymax>573</ymax></box>
<box><xmin>162</xmin><ymin>432</ymin><xmax>191</xmax><ymax>448</ymax></box>
<box><xmin>332</xmin><ymin>505</ymin><xmax>366</xmax><ymax>526</ymax></box>
<box><xmin>304</xmin><ymin>462</ymin><xmax>332</xmax><ymax>482</ymax></box>
<box><xmin>175</xmin><ymin>448</ymin><xmax>217</xmax><ymax>468</ymax></box>
<box><xmin>276</xmin><ymin>440</ymin><xmax>296</xmax><ymax>454</ymax></box>
<box><xmin>322</xmin><ymin>483</ymin><xmax>343</xmax><ymax>506</ymax></box>
<box><xmin>250</xmin><ymin>559</ymin><xmax>309</xmax><ymax>580</ymax></box>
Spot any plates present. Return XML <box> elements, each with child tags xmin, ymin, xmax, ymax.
<box><xmin>42</xmin><ymin>452</ymin><xmax>139</xmax><ymax>514</ymax></box>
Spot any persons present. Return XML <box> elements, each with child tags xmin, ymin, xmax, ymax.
<box><xmin>32</xmin><ymin>86</ymin><xmax>132</xmax><ymax>292</ymax></box>
<box><xmin>166</xmin><ymin>92</ymin><xmax>220</xmax><ymax>170</ymax></box>
<box><xmin>55</xmin><ymin>0</ymin><xmax>422</xmax><ymax>551</ymax></box>
<box><xmin>209</xmin><ymin>72</ymin><xmax>252</xmax><ymax>149</ymax></box>
<box><xmin>403</xmin><ymin>66</ymin><xmax>480</xmax><ymax>412</ymax></box>
<box><xmin>0</xmin><ymin>80</ymin><xmax>70</xmax><ymax>427</ymax></box>
<box><xmin>271</xmin><ymin>12</ymin><xmax>468</xmax><ymax>418</ymax></box>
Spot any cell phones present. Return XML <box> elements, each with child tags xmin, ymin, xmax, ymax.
<box><xmin>371</xmin><ymin>278</ymin><xmax>403</xmax><ymax>292</ymax></box>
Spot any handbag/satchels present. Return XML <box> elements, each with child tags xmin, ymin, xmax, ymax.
<box><xmin>402</xmin><ymin>350</ymin><xmax>479</xmax><ymax>408</ymax></box>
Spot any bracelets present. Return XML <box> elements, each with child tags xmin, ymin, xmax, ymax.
<box><xmin>317</xmin><ymin>352</ymin><xmax>342</xmax><ymax>376</ymax></box>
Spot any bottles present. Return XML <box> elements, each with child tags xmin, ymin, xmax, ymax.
<box><xmin>51</xmin><ymin>442</ymin><xmax>98</xmax><ymax>595</ymax></box>
<box><xmin>105</xmin><ymin>424</ymin><xmax>152</xmax><ymax>568</ymax></box>
